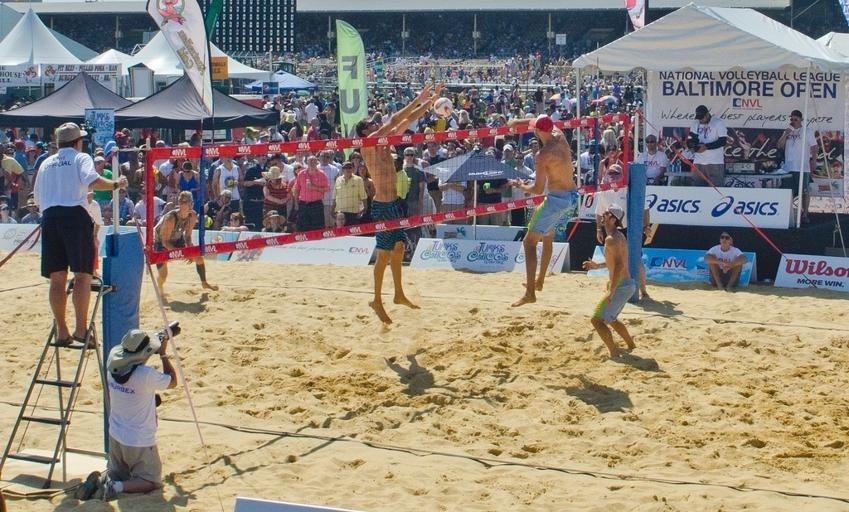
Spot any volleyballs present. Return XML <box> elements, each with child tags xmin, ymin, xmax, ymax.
<box><xmin>434</xmin><ymin>97</ymin><xmax>453</xmax><ymax>118</ymax></box>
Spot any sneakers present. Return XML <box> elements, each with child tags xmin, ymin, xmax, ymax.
<box><xmin>76</xmin><ymin>471</ymin><xmax>118</xmax><ymax>503</ymax></box>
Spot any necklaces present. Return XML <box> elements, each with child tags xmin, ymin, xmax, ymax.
<box><xmin>354</xmin><ymin>80</ymin><xmax>445</xmax><ymax>322</ymax></box>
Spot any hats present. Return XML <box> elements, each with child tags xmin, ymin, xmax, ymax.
<box><xmin>695</xmin><ymin>105</ymin><xmax>708</xmax><ymax>119</ymax></box>
<box><xmin>261</xmin><ymin>165</ymin><xmax>286</xmax><ymax>181</ymax></box>
<box><xmin>55</xmin><ymin>121</ymin><xmax>89</xmax><ymax>143</ymax></box>
<box><xmin>791</xmin><ymin>109</ymin><xmax>803</xmax><ymax>117</ymax></box>
<box><xmin>609</xmin><ymin>203</ymin><xmax>625</xmax><ymax>229</ymax></box>
<box><xmin>263</xmin><ymin>211</ymin><xmax>286</xmax><ymax>228</ymax></box>
<box><xmin>534</xmin><ymin>114</ymin><xmax>553</xmax><ymax>132</ymax></box>
<box><xmin>106</xmin><ymin>328</ymin><xmax>162</xmax><ymax>376</ymax></box>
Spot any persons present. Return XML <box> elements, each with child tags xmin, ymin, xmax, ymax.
<box><xmin>703</xmin><ymin>229</ymin><xmax>747</xmax><ymax>290</ymax></box>
<box><xmin>74</xmin><ymin>328</ymin><xmax>177</xmax><ymax>502</ymax></box>
<box><xmin>34</xmin><ymin>123</ymin><xmax>129</xmax><ymax>344</ymax></box>
<box><xmin>153</xmin><ymin>191</ymin><xmax>217</xmax><ymax>306</ymax></box>
<box><xmin>0</xmin><ymin>0</ymin><xmax>849</xmax><ymax>277</ymax></box>
<box><xmin>583</xmin><ymin>205</ymin><xmax>638</xmax><ymax>359</ymax></box>
<box><xmin>594</xmin><ymin>165</ymin><xmax>651</xmax><ymax>302</ymax></box>
<box><xmin>505</xmin><ymin>114</ymin><xmax>579</xmax><ymax>307</ymax></box>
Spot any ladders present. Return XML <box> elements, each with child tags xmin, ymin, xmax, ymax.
<box><xmin>0</xmin><ymin>274</ymin><xmax>117</xmax><ymax>489</ymax></box>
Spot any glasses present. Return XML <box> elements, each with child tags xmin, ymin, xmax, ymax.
<box><xmin>720</xmin><ymin>235</ymin><xmax>730</xmax><ymax>239</ymax></box>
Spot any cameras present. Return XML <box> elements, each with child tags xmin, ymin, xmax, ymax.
<box><xmin>155</xmin><ymin>321</ymin><xmax>182</xmax><ymax>343</ymax></box>
